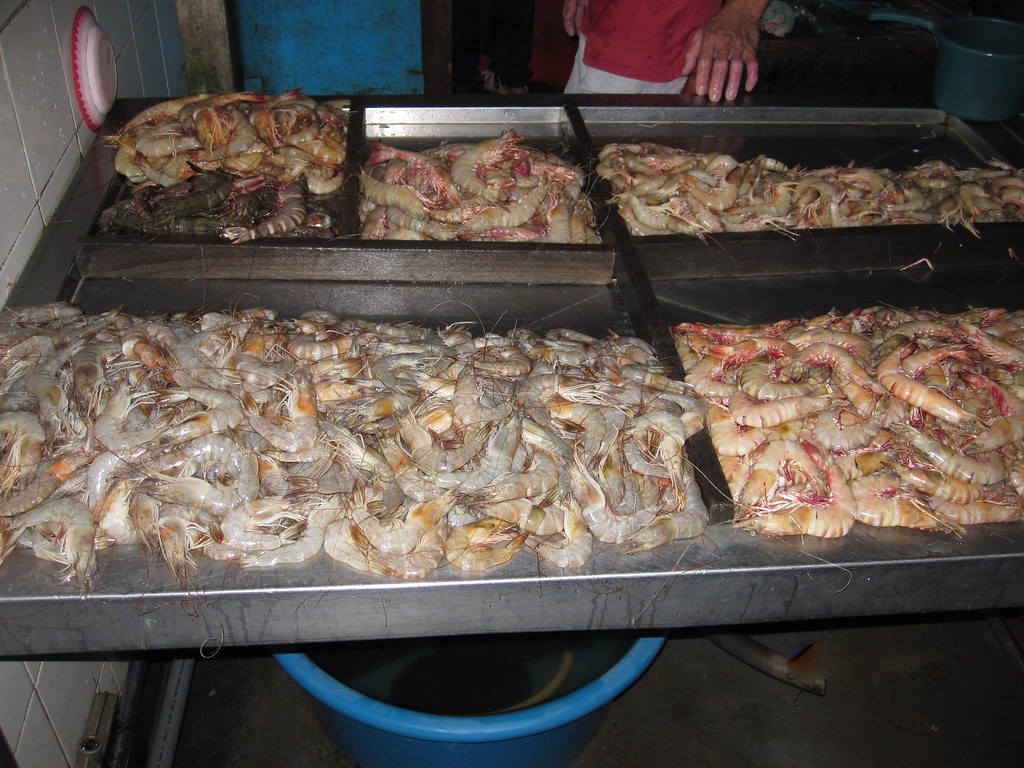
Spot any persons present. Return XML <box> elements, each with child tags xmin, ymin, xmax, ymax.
<box><xmin>562</xmin><ymin>0</ymin><xmax>770</xmax><ymax>103</ymax></box>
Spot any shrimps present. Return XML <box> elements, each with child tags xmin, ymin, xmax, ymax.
<box><xmin>597</xmin><ymin>136</ymin><xmax>1024</xmax><ymax>243</ymax></box>
<box><xmin>104</xmin><ymin>85</ymin><xmax>349</xmax><ymax>242</ymax></box>
<box><xmin>673</xmin><ymin>304</ymin><xmax>1024</xmax><ymax>543</ymax></box>
<box><xmin>355</xmin><ymin>127</ymin><xmax>593</xmax><ymax>242</ymax></box>
<box><xmin>3</xmin><ymin>291</ymin><xmax>711</xmax><ymax>583</ymax></box>
